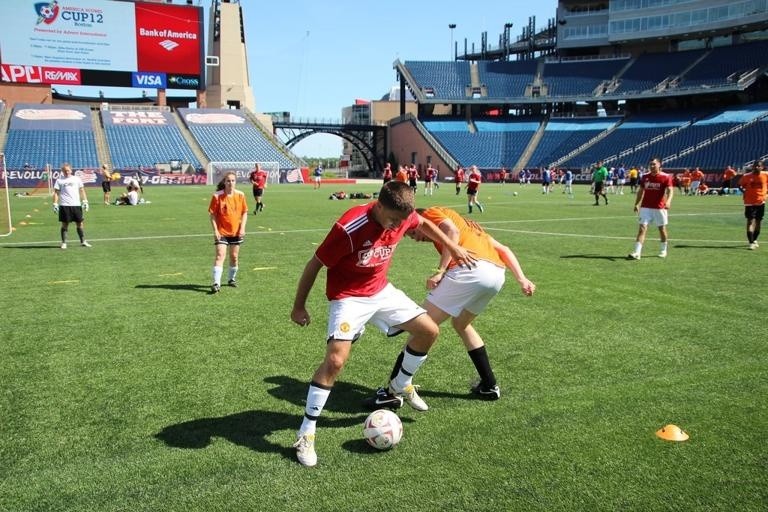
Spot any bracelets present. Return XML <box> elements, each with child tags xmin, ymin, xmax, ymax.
<box><xmin>435</xmin><ymin>269</ymin><xmax>445</xmax><ymax>273</ymax></box>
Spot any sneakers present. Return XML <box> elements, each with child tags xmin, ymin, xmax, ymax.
<box><xmin>61</xmin><ymin>242</ymin><xmax>92</xmax><ymax>249</ymax></box>
<box><xmin>211</xmin><ymin>279</ymin><xmax>237</xmax><ymax>293</ymax></box>
<box><xmin>364</xmin><ymin>388</ymin><xmax>404</xmax><ymax>410</ymax></box>
<box><xmin>658</xmin><ymin>250</ymin><xmax>667</xmax><ymax>257</ymax></box>
<box><xmin>748</xmin><ymin>240</ymin><xmax>759</xmax><ymax>249</ymax></box>
<box><xmin>295</xmin><ymin>430</ymin><xmax>317</xmax><ymax>466</ymax></box>
<box><xmin>628</xmin><ymin>252</ymin><xmax>640</xmax><ymax>260</ymax></box>
<box><xmin>387</xmin><ymin>379</ymin><xmax>428</xmax><ymax>411</ymax></box>
<box><xmin>471</xmin><ymin>383</ymin><xmax>500</xmax><ymax>400</ymax></box>
<box><xmin>253</xmin><ymin>204</ymin><xmax>265</xmax><ymax>215</ymax></box>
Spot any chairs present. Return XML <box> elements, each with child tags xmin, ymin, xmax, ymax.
<box><xmin>405</xmin><ymin>39</ymin><xmax>768</xmax><ymax>173</ymax></box>
<box><xmin>2</xmin><ymin>102</ymin><xmax>298</xmax><ymax>171</ymax></box>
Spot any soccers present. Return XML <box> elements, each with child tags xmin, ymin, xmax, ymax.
<box><xmin>363</xmin><ymin>409</ymin><xmax>404</xmax><ymax>450</ymax></box>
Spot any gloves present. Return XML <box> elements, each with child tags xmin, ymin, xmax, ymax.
<box><xmin>82</xmin><ymin>200</ymin><xmax>88</xmax><ymax>211</ymax></box>
<box><xmin>52</xmin><ymin>203</ymin><xmax>59</xmax><ymax>213</ymax></box>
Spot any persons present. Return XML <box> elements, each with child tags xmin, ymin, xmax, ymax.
<box><xmin>329</xmin><ymin>191</ymin><xmax>379</xmax><ymax>200</ymax></box>
<box><xmin>383</xmin><ymin>162</ymin><xmax>419</xmax><ymax>194</ymax></box>
<box><xmin>250</xmin><ymin>163</ymin><xmax>268</xmax><ymax>214</ymax></box>
<box><xmin>362</xmin><ymin>208</ymin><xmax>536</xmax><ymax>410</ymax></box>
<box><xmin>591</xmin><ymin>162</ymin><xmax>649</xmax><ymax>206</ymax></box>
<box><xmin>24</xmin><ymin>163</ymin><xmax>29</xmax><ymax>168</ymax></box>
<box><xmin>454</xmin><ymin>165</ymin><xmax>483</xmax><ymax>214</ymax></box>
<box><xmin>291</xmin><ymin>180</ymin><xmax>478</xmax><ymax>467</ymax></box>
<box><xmin>518</xmin><ymin>168</ymin><xmax>532</xmax><ymax>188</ymax></box>
<box><xmin>542</xmin><ymin>165</ymin><xmax>573</xmax><ymax>195</ymax></box>
<box><xmin>424</xmin><ymin>163</ymin><xmax>439</xmax><ymax>195</ymax></box>
<box><xmin>676</xmin><ymin>165</ymin><xmax>735</xmax><ymax>196</ymax></box>
<box><xmin>53</xmin><ymin>164</ymin><xmax>92</xmax><ymax>248</ymax></box>
<box><xmin>208</xmin><ymin>171</ymin><xmax>248</xmax><ymax>292</ymax></box>
<box><xmin>629</xmin><ymin>159</ymin><xmax>673</xmax><ymax>260</ymax></box>
<box><xmin>101</xmin><ymin>163</ymin><xmax>148</xmax><ymax>206</ymax></box>
<box><xmin>737</xmin><ymin>160</ymin><xmax>768</xmax><ymax>249</ymax></box>
<box><xmin>499</xmin><ymin>167</ymin><xmax>506</xmax><ymax>184</ymax></box>
<box><xmin>314</xmin><ymin>164</ymin><xmax>322</xmax><ymax>189</ymax></box>
